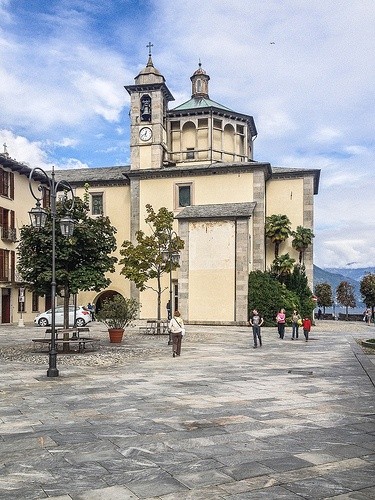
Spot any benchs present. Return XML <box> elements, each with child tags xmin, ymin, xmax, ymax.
<box><xmin>139</xmin><ymin>320</ymin><xmax>171</xmax><ymax>335</ymax></box>
<box><xmin>32</xmin><ymin>328</ymin><xmax>101</xmax><ymax>354</ymax></box>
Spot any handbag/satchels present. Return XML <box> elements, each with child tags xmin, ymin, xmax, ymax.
<box><xmin>297</xmin><ymin>319</ymin><xmax>302</xmax><ymax>326</ymax></box>
<box><xmin>181</xmin><ymin>328</ymin><xmax>186</xmax><ymax>335</ymax></box>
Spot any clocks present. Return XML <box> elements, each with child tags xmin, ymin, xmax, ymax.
<box><xmin>139</xmin><ymin>127</ymin><xmax>152</xmax><ymax>141</ymax></box>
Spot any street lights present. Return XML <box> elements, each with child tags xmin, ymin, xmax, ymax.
<box><xmin>27</xmin><ymin>165</ymin><xmax>76</xmax><ymax>377</ymax></box>
<box><xmin>160</xmin><ymin>231</ymin><xmax>180</xmax><ymax>321</ymax></box>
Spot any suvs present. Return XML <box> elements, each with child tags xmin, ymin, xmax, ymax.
<box><xmin>34</xmin><ymin>306</ymin><xmax>93</xmax><ymax>327</ymax></box>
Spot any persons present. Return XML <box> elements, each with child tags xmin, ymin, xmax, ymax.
<box><xmin>302</xmin><ymin>316</ymin><xmax>311</xmax><ymax>342</ymax></box>
<box><xmin>169</xmin><ymin>311</ymin><xmax>184</xmax><ymax>357</ymax></box>
<box><xmin>276</xmin><ymin>308</ymin><xmax>286</xmax><ymax>339</ymax></box>
<box><xmin>317</xmin><ymin>309</ymin><xmax>321</xmax><ymax>320</ymax></box>
<box><xmin>88</xmin><ymin>303</ymin><xmax>96</xmax><ymax>321</ymax></box>
<box><xmin>291</xmin><ymin>309</ymin><xmax>302</xmax><ymax>340</ymax></box>
<box><xmin>250</xmin><ymin>308</ymin><xmax>264</xmax><ymax>348</ymax></box>
<box><xmin>364</xmin><ymin>307</ymin><xmax>372</xmax><ymax>326</ymax></box>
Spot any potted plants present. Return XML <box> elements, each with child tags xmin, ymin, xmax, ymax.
<box><xmin>95</xmin><ymin>294</ymin><xmax>142</xmax><ymax>343</ymax></box>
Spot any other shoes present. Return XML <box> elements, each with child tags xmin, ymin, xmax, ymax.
<box><xmin>291</xmin><ymin>337</ymin><xmax>294</xmax><ymax>340</ymax></box>
<box><xmin>253</xmin><ymin>346</ymin><xmax>257</xmax><ymax>348</ymax></box>
<box><xmin>176</xmin><ymin>352</ymin><xmax>180</xmax><ymax>356</ymax></box>
<box><xmin>260</xmin><ymin>344</ymin><xmax>262</xmax><ymax>347</ymax></box>
<box><xmin>296</xmin><ymin>338</ymin><xmax>298</xmax><ymax>340</ymax></box>
<box><xmin>173</xmin><ymin>353</ymin><xmax>175</xmax><ymax>357</ymax></box>
